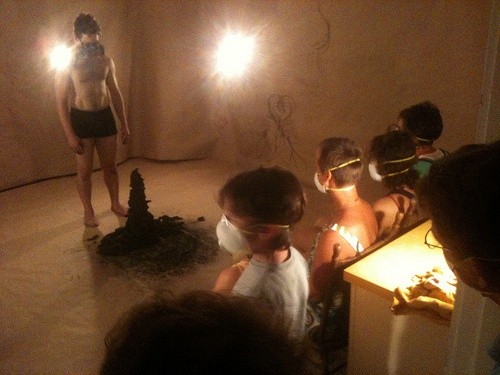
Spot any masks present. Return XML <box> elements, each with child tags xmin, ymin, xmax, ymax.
<box><xmin>368</xmin><ymin>162</ymin><xmax>382</xmax><ymax>182</ymax></box>
<box><xmin>216</xmin><ymin>216</ymin><xmax>250</xmax><ymax>256</ymax></box>
<box><xmin>314</xmin><ymin>172</ymin><xmax>328</xmax><ymax>194</ymax></box>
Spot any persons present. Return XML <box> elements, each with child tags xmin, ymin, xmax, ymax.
<box><xmin>99</xmin><ymin>289</ymin><xmax>307</xmax><ymax>375</ymax></box>
<box><xmin>399</xmin><ymin>100</ymin><xmax>449</xmax><ymax>180</ymax></box>
<box><xmin>305</xmin><ymin>137</ymin><xmax>378</xmax><ymax>325</ymax></box>
<box><xmin>424</xmin><ymin>140</ymin><xmax>500</xmax><ymax>375</ymax></box>
<box><xmin>56</xmin><ymin>12</ymin><xmax>131</xmax><ymax>227</ymax></box>
<box><xmin>367</xmin><ymin>124</ymin><xmax>429</xmax><ymax>243</ymax></box>
<box><xmin>214</xmin><ymin>167</ymin><xmax>310</xmax><ymax>342</ymax></box>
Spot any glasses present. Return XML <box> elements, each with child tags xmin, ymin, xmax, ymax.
<box><xmin>79</xmin><ymin>38</ymin><xmax>99</xmax><ymax>47</ymax></box>
<box><xmin>424</xmin><ymin>228</ymin><xmax>492</xmax><ymax>268</ymax></box>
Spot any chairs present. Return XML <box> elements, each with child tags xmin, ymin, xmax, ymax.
<box><xmin>307</xmin><ymin>212</ymin><xmax>405</xmax><ymax>375</ymax></box>
<box><xmin>398</xmin><ymin>198</ymin><xmax>432</xmax><ymax>237</ymax></box>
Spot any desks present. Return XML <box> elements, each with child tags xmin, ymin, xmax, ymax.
<box><xmin>342</xmin><ymin>218</ymin><xmax>459</xmax><ymax>375</ymax></box>
